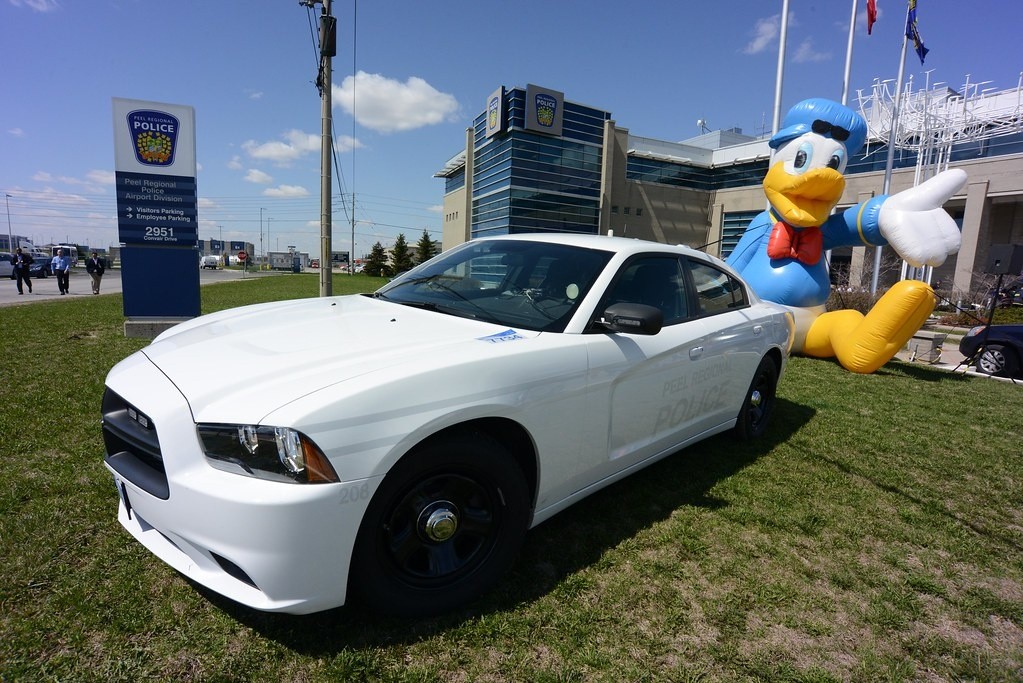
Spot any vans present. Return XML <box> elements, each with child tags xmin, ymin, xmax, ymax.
<box><xmin>52</xmin><ymin>246</ymin><xmax>79</xmax><ymax>267</ymax></box>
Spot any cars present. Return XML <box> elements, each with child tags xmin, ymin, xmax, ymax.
<box><xmin>1</xmin><ymin>252</ymin><xmax>25</xmax><ymax>280</ymax></box>
<box><xmin>959</xmin><ymin>323</ymin><xmax>1023</xmax><ymax>375</ymax></box>
<box><xmin>200</xmin><ymin>256</ymin><xmax>217</xmax><ymax>269</ymax></box>
<box><xmin>25</xmin><ymin>257</ymin><xmax>55</xmax><ymax>278</ymax></box>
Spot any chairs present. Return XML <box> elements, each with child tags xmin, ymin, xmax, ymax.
<box><xmin>537</xmin><ymin>256</ymin><xmax>585</xmax><ymax>300</ymax></box>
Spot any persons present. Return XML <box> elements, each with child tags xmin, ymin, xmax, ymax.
<box><xmin>931</xmin><ymin>280</ymin><xmax>941</xmax><ymax>290</ymax></box>
<box><xmin>51</xmin><ymin>250</ymin><xmax>70</xmax><ymax>295</ymax></box>
<box><xmin>11</xmin><ymin>248</ymin><xmax>34</xmax><ymax>295</ymax></box>
<box><xmin>86</xmin><ymin>253</ymin><xmax>105</xmax><ymax>295</ymax></box>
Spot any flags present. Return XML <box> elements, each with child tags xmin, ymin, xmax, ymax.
<box><xmin>906</xmin><ymin>0</ymin><xmax>929</xmax><ymax>65</ymax></box>
<box><xmin>867</xmin><ymin>0</ymin><xmax>878</xmax><ymax>35</ymax></box>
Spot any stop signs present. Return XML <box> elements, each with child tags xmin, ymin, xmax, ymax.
<box><xmin>238</xmin><ymin>251</ymin><xmax>247</xmax><ymax>259</ymax></box>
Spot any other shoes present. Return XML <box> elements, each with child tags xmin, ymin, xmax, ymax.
<box><xmin>29</xmin><ymin>286</ymin><xmax>32</xmax><ymax>293</ymax></box>
<box><xmin>61</xmin><ymin>292</ymin><xmax>65</xmax><ymax>295</ymax></box>
<box><xmin>94</xmin><ymin>292</ymin><xmax>99</xmax><ymax>295</ymax></box>
<box><xmin>66</xmin><ymin>289</ymin><xmax>69</xmax><ymax>293</ymax></box>
<box><xmin>18</xmin><ymin>292</ymin><xmax>23</xmax><ymax>295</ymax></box>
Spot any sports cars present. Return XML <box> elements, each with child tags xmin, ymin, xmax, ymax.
<box><xmin>99</xmin><ymin>232</ymin><xmax>795</xmax><ymax>617</ymax></box>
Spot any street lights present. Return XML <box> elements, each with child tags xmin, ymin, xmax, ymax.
<box><xmin>6</xmin><ymin>194</ymin><xmax>12</xmax><ymax>254</ymax></box>
<box><xmin>259</xmin><ymin>207</ymin><xmax>267</xmax><ymax>265</ymax></box>
<box><xmin>218</xmin><ymin>225</ymin><xmax>224</xmax><ymax>257</ymax></box>
